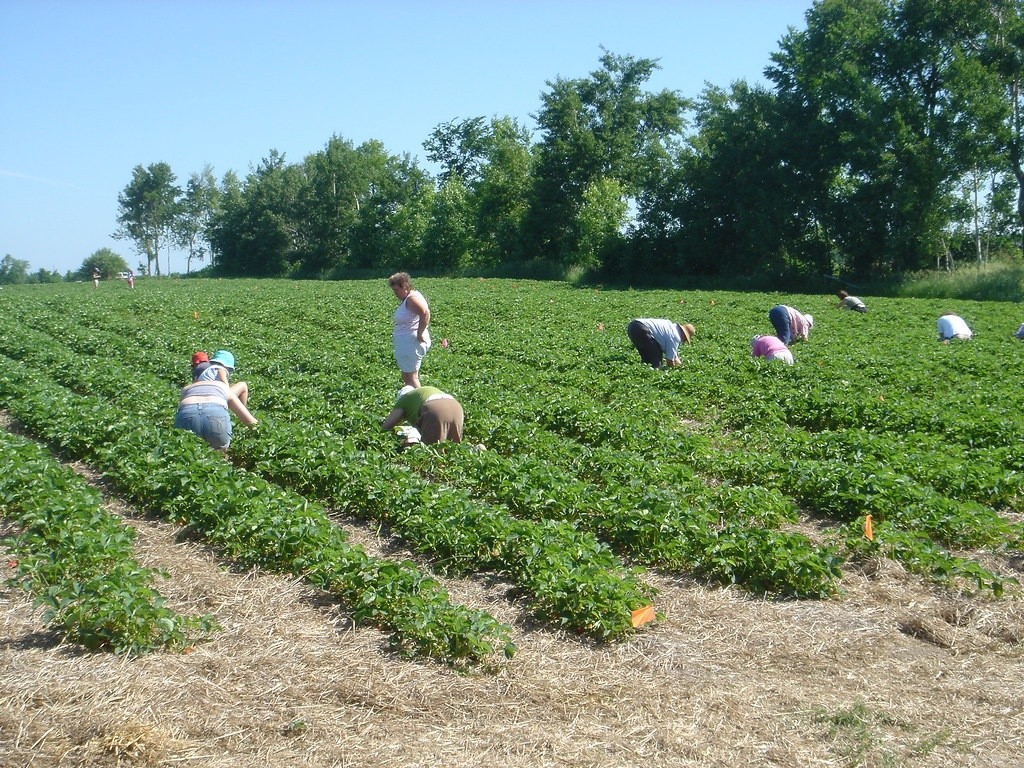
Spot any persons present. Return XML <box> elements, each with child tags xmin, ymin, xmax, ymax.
<box><xmin>750</xmin><ymin>334</ymin><xmax>796</xmax><ymax>365</ymax></box>
<box><xmin>628</xmin><ymin>318</ymin><xmax>695</xmax><ymax>371</ymax></box>
<box><xmin>836</xmin><ymin>290</ymin><xmax>865</xmax><ymax>314</ymax></box>
<box><xmin>388</xmin><ymin>272</ymin><xmax>432</xmax><ymax>389</ymax></box>
<box><xmin>173</xmin><ymin>349</ymin><xmax>259</xmax><ymax>459</ymax></box>
<box><xmin>937</xmin><ymin>310</ymin><xmax>974</xmax><ymax>345</ymax></box>
<box><xmin>769</xmin><ymin>304</ymin><xmax>814</xmax><ymax>347</ymax></box>
<box><xmin>378</xmin><ymin>385</ymin><xmax>463</xmax><ymax>449</ymax></box>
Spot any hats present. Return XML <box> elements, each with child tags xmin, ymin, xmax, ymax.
<box><xmin>191</xmin><ymin>351</ymin><xmax>210</xmax><ymax>365</ymax></box>
<box><xmin>95</xmin><ymin>268</ymin><xmax>99</xmax><ymax>272</ymax></box>
<box><xmin>209</xmin><ymin>350</ymin><xmax>235</xmax><ymax>372</ymax></box>
<box><xmin>804</xmin><ymin>314</ymin><xmax>814</xmax><ymax>327</ymax></box>
<box><xmin>676</xmin><ymin>320</ymin><xmax>695</xmax><ymax>345</ymax></box>
<box><xmin>397</xmin><ymin>384</ymin><xmax>414</xmax><ymax>399</ymax></box>
<box><xmin>750</xmin><ymin>335</ymin><xmax>761</xmax><ymax>347</ymax></box>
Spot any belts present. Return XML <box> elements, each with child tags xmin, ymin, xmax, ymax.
<box><xmin>425</xmin><ymin>393</ymin><xmax>455</xmax><ymax>402</ymax></box>
